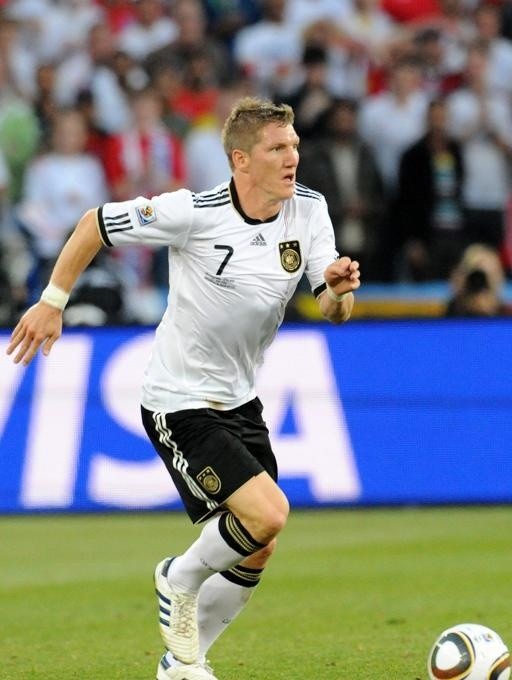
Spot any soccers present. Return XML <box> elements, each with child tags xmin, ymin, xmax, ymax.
<box><xmin>428</xmin><ymin>623</ymin><xmax>511</xmax><ymax>680</ymax></box>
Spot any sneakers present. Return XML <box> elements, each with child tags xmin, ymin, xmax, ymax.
<box><xmin>155</xmin><ymin>649</ymin><xmax>219</xmax><ymax>680</ymax></box>
<box><xmin>152</xmin><ymin>555</ymin><xmax>200</xmax><ymax>666</ymax></box>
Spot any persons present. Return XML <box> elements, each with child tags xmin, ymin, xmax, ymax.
<box><xmin>4</xmin><ymin>95</ymin><xmax>363</xmax><ymax>680</ymax></box>
<box><xmin>0</xmin><ymin>1</ymin><xmax>511</xmax><ymax>321</ymax></box>
